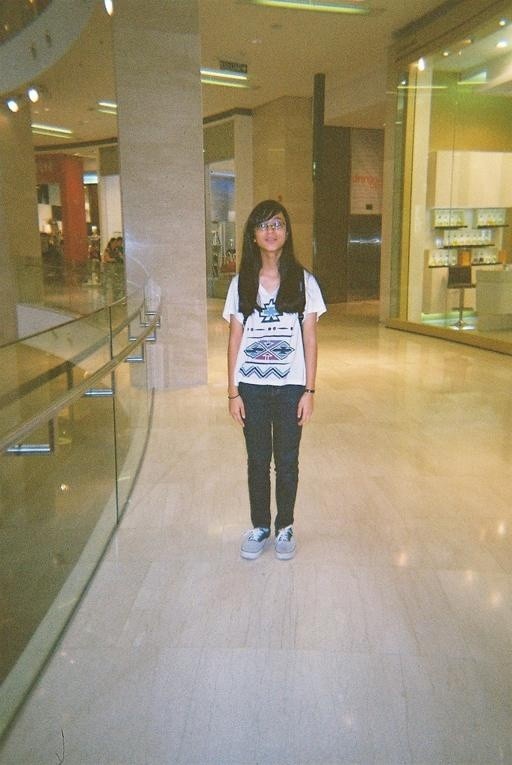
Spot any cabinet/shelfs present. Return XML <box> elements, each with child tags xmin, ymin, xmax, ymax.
<box><xmin>429</xmin><ymin>224</ymin><xmax>509</xmax><ymax>268</ymax></box>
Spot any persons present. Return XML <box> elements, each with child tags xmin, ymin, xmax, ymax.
<box><xmin>102</xmin><ymin>236</ymin><xmax>124</xmax><ymax>263</ymax></box>
<box><xmin>116</xmin><ymin>237</ymin><xmax>123</xmax><ymax>255</ymax></box>
<box><xmin>221</xmin><ymin>200</ymin><xmax>327</xmax><ymax>561</ymax></box>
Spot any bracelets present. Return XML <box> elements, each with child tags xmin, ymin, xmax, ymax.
<box><xmin>304</xmin><ymin>388</ymin><xmax>316</xmax><ymax>393</ymax></box>
<box><xmin>227</xmin><ymin>394</ymin><xmax>241</xmax><ymax>400</ymax></box>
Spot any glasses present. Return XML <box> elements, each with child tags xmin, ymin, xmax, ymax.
<box><xmin>255</xmin><ymin>220</ymin><xmax>287</xmax><ymax>231</ymax></box>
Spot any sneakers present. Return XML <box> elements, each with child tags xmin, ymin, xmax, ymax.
<box><xmin>240</xmin><ymin>527</ymin><xmax>271</xmax><ymax>560</ymax></box>
<box><xmin>275</xmin><ymin>524</ymin><xmax>297</xmax><ymax>559</ymax></box>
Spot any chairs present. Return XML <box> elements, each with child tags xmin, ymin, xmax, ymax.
<box><xmin>448</xmin><ymin>266</ymin><xmax>476</xmax><ymax>330</ymax></box>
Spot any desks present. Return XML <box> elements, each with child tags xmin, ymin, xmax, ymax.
<box><xmin>476</xmin><ymin>270</ymin><xmax>512</xmax><ymax>331</ymax></box>
